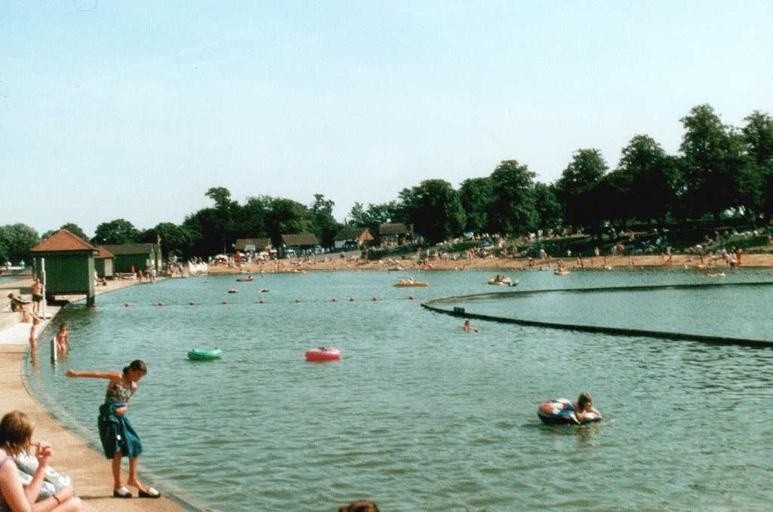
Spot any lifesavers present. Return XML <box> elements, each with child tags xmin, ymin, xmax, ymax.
<box><xmin>13</xmin><ymin>453</ymin><xmax>66</xmax><ymax>501</ymax></box>
<box><xmin>536</xmin><ymin>398</ymin><xmax>601</xmax><ymax>424</ymax></box>
<box><xmin>187</xmin><ymin>346</ymin><xmax>222</xmax><ymax>360</ymax></box>
<box><xmin>304</xmin><ymin>348</ymin><xmax>340</xmax><ymax>361</ymax></box>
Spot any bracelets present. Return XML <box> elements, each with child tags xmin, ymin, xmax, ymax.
<box><xmin>51</xmin><ymin>495</ymin><xmax>61</xmax><ymax>506</ymax></box>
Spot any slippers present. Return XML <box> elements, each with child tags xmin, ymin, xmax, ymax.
<box><xmin>138</xmin><ymin>487</ymin><xmax>160</xmax><ymax>498</ymax></box>
<box><xmin>113</xmin><ymin>486</ymin><xmax>131</xmax><ymax>498</ymax></box>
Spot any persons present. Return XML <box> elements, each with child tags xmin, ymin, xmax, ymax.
<box><xmin>592</xmin><ymin>230</ymin><xmax>743</xmax><ymax>277</ymax></box>
<box><xmin>362</xmin><ymin>230</ymin><xmax>584</xmax><ymax>285</ymax></box>
<box><xmin>63</xmin><ymin>359</ymin><xmax>161</xmax><ymax>497</ymax></box>
<box><xmin>138</xmin><ymin>235</ymin><xmax>360</xmax><ymax>284</ymax></box>
<box><xmin>0</xmin><ymin>257</ymin><xmax>43</xmax><ymax>347</ymax></box>
<box><xmin>0</xmin><ymin>410</ymin><xmax>83</xmax><ymax>512</ymax></box>
<box><xmin>56</xmin><ymin>320</ymin><xmax>68</xmax><ymax>361</ymax></box>
<box><xmin>574</xmin><ymin>391</ymin><xmax>600</xmax><ymax>422</ymax></box>
<box><xmin>463</xmin><ymin>320</ymin><xmax>474</xmax><ymax>335</ymax></box>
